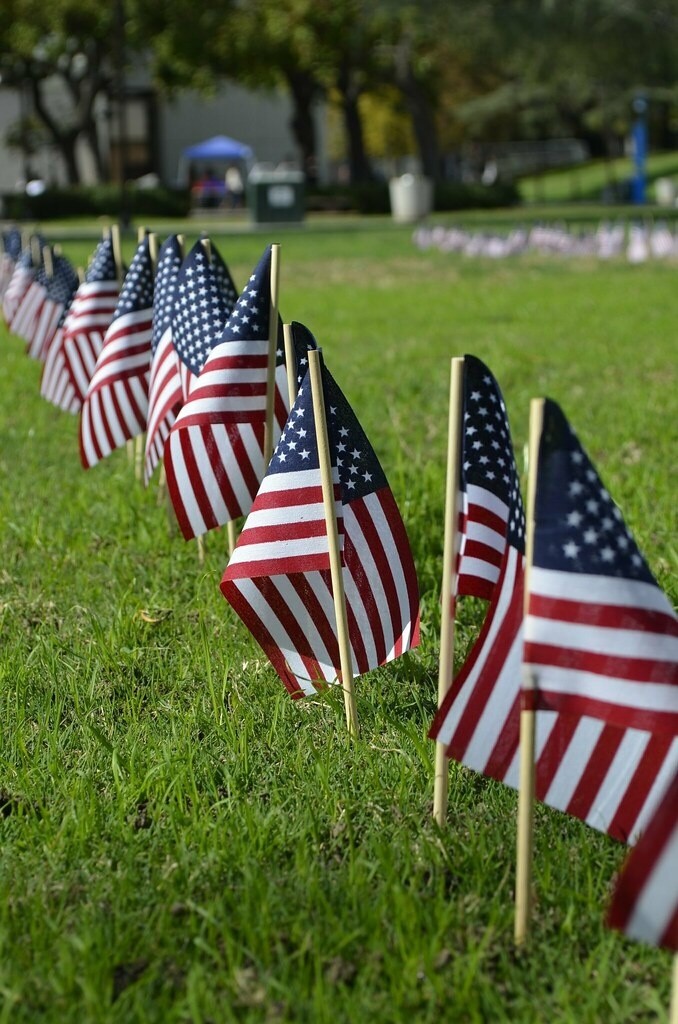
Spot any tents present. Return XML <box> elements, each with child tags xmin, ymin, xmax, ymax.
<box><xmin>174</xmin><ymin>136</ymin><xmax>254</xmax><ymax>195</ymax></box>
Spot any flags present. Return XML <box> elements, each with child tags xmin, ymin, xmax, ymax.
<box><xmin>0</xmin><ymin>217</ymin><xmax>243</xmax><ymax>490</ymax></box>
<box><xmin>220</xmin><ymin>346</ymin><xmax>423</xmax><ymax>700</ymax></box>
<box><xmin>438</xmin><ymin>352</ymin><xmax>518</xmax><ymax>609</ymax></box>
<box><xmin>289</xmin><ymin>321</ymin><xmax>319</xmax><ymax>401</ymax></box>
<box><xmin>162</xmin><ymin>242</ymin><xmax>292</xmax><ymax>542</ymax></box>
<box><xmin>421</xmin><ymin>394</ymin><xmax>678</xmax><ymax>953</ymax></box>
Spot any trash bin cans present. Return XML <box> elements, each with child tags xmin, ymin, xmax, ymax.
<box><xmin>247</xmin><ymin>162</ymin><xmax>304</xmax><ymax>223</ymax></box>
<box><xmin>389</xmin><ymin>174</ymin><xmax>432</xmax><ymax>222</ymax></box>
